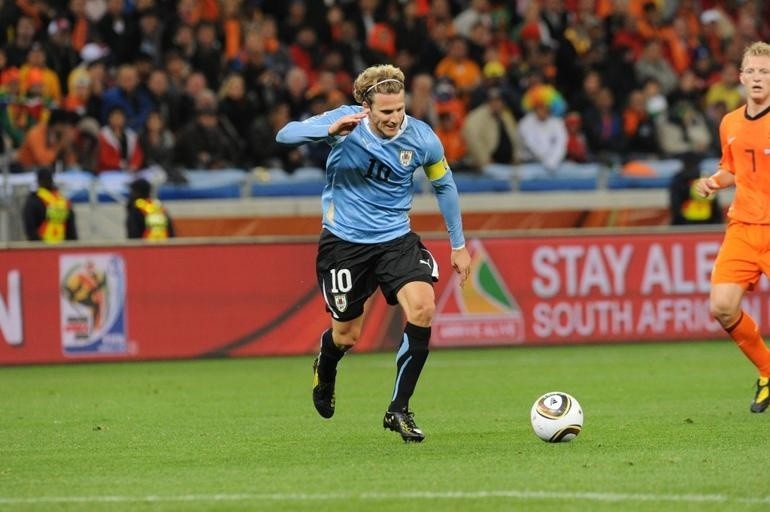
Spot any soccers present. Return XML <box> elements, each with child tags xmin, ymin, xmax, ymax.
<box><xmin>531</xmin><ymin>391</ymin><xmax>583</xmax><ymax>442</ymax></box>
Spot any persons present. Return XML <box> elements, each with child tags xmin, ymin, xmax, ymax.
<box><xmin>20</xmin><ymin>167</ymin><xmax>79</xmax><ymax>241</ymax></box>
<box><xmin>126</xmin><ymin>179</ymin><xmax>176</xmax><ymax>243</ymax></box>
<box><xmin>0</xmin><ymin>0</ymin><xmax>770</xmax><ymax>183</ymax></box>
<box><xmin>668</xmin><ymin>150</ymin><xmax>722</xmax><ymax>226</ymax></box>
<box><xmin>694</xmin><ymin>41</ymin><xmax>770</xmax><ymax>412</ymax></box>
<box><xmin>275</xmin><ymin>64</ymin><xmax>471</xmax><ymax>443</ymax></box>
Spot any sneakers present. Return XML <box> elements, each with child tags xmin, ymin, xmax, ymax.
<box><xmin>383</xmin><ymin>408</ymin><xmax>425</xmax><ymax>442</ymax></box>
<box><xmin>751</xmin><ymin>376</ymin><xmax>770</xmax><ymax>413</ymax></box>
<box><xmin>313</xmin><ymin>351</ymin><xmax>337</xmax><ymax>418</ymax></box>
